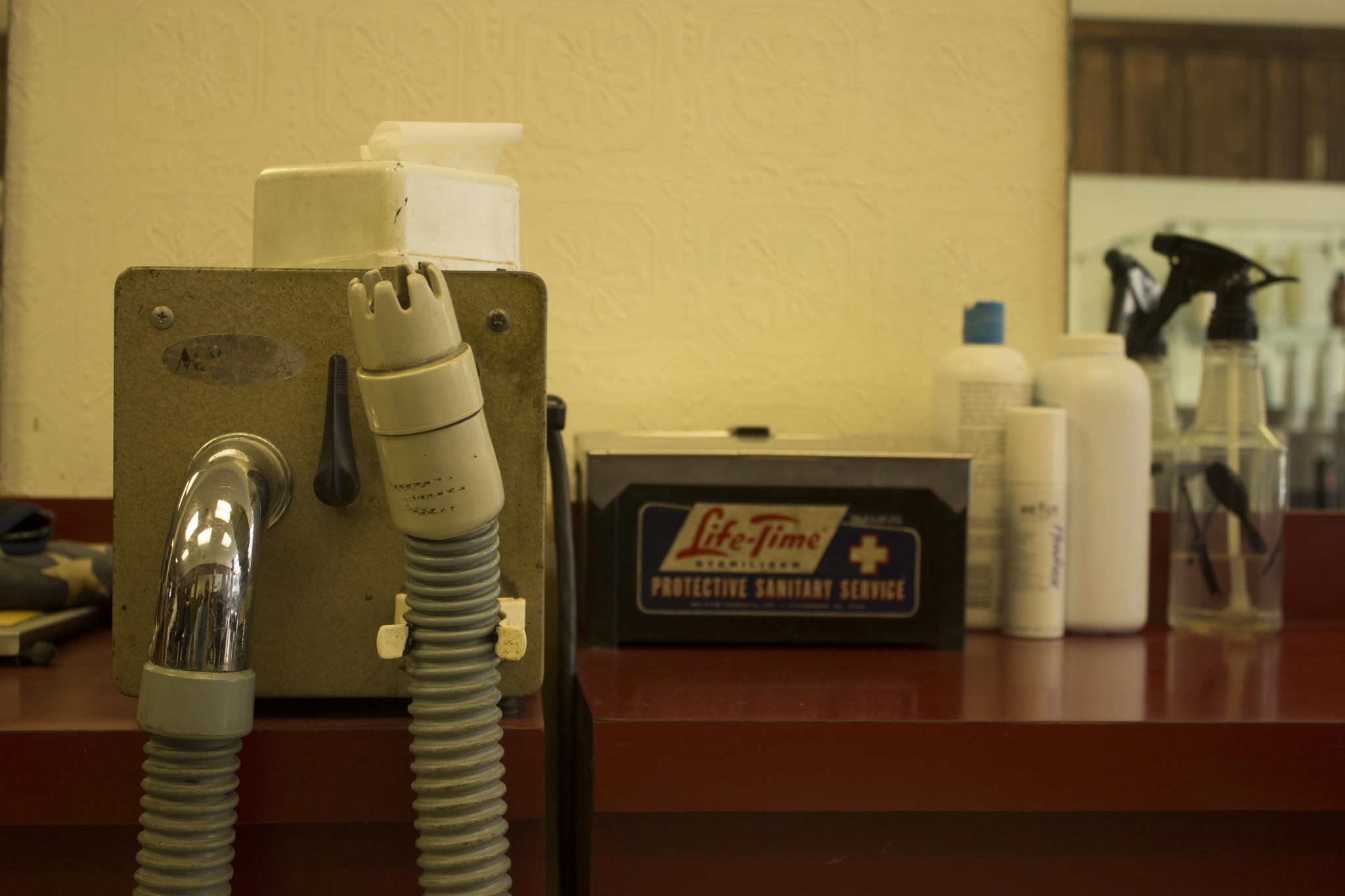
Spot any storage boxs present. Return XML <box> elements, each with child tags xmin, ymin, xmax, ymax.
<box><xmin>573</xmin><ymin>426</ymin><xmax>970</xmax><ymax>656</ymax></box>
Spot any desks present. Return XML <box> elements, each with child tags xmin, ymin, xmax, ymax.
<box><xmin>0</xmin><ymin>626</ymin><xmax>548</xmax><ymax>895</ymax></box>
<box><xmin>555</xmin><ymin>601</ymin><xmax>1345</xmax><ymax>896</ymax></box>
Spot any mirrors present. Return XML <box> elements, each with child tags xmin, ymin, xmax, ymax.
<box><xmin>1063</xmin><ymin>172</ymin><xmax>1345</xmax><ymax>517</ymax></box>
